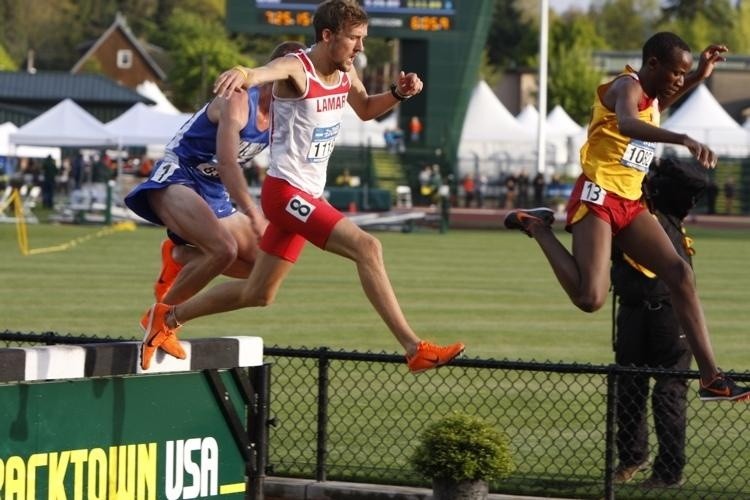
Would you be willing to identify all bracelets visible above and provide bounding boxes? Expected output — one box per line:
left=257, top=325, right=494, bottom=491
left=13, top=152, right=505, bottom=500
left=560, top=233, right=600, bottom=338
left=388, top=83, right=412, bottom=103
left=232, top=65, right=250, bottom=80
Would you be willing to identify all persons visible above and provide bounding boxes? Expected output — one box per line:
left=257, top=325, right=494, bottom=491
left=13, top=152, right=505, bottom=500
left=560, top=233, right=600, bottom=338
left=336, top=167, right=361, bottom=189
left=0, top=150, right=153, bottom=210
left=705, top=174, right=735, bottom=217
left=382, top=116, right=425, bottom=156
left=417, top=161, right=562, bottom=211
left=241, top=160, right=266, bottom=188
left=609, top=156, right=711, bottom=492
left=124, top=41, right=309, bottom=333
left=503, top=32, right=749, bottom=403
left=140, top=1, right=466, bottom=374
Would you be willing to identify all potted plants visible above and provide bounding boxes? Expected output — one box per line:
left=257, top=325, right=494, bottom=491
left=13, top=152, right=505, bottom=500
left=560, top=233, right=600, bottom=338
left=403, top=410, right=521, bottom=500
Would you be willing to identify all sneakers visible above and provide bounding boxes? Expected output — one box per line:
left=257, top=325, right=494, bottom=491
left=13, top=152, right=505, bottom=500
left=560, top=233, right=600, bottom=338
left=612, top=451, right=653, bottom=484
left=504, top=206, right=556, bottom=238
left=154, top=236, right=183, bottom=302
left=638, top=476, right=681, bottom=492
left=698, top=374, right=750, bottom=402
left=140, top=302, right=188, bottom=371
left=406, top=340, right=465, bottom=376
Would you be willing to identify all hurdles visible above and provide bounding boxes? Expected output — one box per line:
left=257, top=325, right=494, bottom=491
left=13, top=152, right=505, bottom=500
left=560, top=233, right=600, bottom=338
left=0, top=334, right=270, bottom=500
